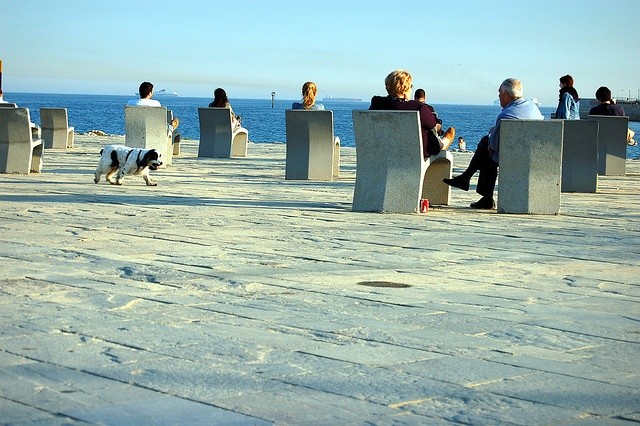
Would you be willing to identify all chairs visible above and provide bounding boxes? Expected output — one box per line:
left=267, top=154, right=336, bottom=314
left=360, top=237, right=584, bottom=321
left=198, top=107, right=248, bottom=158
left=588, top=114, right=629, bottom=177
left=285, top=110, right=341, bottom=182
left=0, top=107, right=44, bottom=175
left=349, top=109, right=454, bottom=214
left=40, top=107, right=74, bottom=149
left=124, top=105, right=172, bottom=166
left=560, top=118, right=599, bottom=194
left=497, top=118, right=565, bottom=216
left=1, top=103, right=41, bottom=141
left=167, top=110, right=181, bottom=156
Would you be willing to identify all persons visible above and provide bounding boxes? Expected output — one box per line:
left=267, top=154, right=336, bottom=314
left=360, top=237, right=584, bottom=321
left=443, top=77, right=545, bottom=209
left=292, top=81, right=325, bottom=110
left=589, top=87, right=638, bottom=147
left=368, top=70, right=455, bottom=157
left=458, top=137, right=466, bottom=150
left=208, top=88, right=241, bottom=132
left=414, top=89, right=444, bottom=136
left=556, top=75, right=580, bottom=120
left=127, top=82, right=179, bottom=134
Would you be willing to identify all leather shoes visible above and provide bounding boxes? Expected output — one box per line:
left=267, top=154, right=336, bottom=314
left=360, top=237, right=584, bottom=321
left=443, top=177, right=469, bottom=191
left=470, top=198, right=495, bottom=208
left=441, top=127, right=454, bottom=150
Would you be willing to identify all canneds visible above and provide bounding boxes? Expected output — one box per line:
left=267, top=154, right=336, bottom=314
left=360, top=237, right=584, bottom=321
left=421, top=199, right=429, bottom=213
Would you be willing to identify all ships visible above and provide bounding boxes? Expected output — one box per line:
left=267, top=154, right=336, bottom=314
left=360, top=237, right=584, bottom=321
left=568, top=94, right=634, bottom=127
left=322, top=93, right=365, bottom=108
left=135, top=88, right=178, bottom=96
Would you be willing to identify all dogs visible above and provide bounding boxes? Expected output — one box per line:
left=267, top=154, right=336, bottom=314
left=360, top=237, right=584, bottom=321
left=94, top=144, right=163, bottom=185
